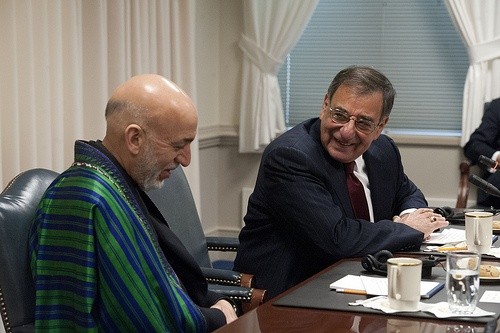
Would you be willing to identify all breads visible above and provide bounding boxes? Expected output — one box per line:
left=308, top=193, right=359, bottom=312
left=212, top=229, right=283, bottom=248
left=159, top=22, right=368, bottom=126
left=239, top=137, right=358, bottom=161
left=493, top=221, right=500, bottom=229
left=479, top=264, right=500, bottom=277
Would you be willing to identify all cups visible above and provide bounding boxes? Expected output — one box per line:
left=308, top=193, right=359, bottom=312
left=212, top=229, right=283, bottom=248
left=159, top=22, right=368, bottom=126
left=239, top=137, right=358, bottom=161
left=445, top=250, right=481, bottom=315
left=465, top=212, right=493, bottom=254
left=387, top=258, right=422, bottom=311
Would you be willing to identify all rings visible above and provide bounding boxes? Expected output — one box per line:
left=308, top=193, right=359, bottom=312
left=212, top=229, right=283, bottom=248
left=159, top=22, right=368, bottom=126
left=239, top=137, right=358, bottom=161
left=429, top=216, right=437, bottom=222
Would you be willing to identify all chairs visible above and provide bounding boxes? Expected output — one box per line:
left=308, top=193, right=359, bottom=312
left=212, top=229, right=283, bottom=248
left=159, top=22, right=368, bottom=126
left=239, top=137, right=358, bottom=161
left=455, top=162, right=479, bottom=209
left=0, top=167, right=271, bottom=333
left=143, top=161, right=257, bottom=288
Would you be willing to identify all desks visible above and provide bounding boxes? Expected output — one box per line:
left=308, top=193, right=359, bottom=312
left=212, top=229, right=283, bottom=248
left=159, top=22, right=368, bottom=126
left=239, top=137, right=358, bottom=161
left=212, top=208, right=500, bottom=333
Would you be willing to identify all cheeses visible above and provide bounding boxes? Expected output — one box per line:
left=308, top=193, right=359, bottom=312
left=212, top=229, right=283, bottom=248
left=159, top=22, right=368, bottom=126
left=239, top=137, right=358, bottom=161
left=439, top=242, right=467, bottom=251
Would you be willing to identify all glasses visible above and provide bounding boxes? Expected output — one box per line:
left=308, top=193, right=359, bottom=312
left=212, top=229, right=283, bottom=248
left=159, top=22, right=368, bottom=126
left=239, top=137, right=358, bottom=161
left=328, top=100, right=383, bottom=135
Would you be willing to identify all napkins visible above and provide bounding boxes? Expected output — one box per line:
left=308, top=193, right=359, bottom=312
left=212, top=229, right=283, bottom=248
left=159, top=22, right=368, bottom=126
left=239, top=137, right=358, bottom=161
left=421, top=301, right=497, bottom=319
left=348, top=294, right=427, bottom=314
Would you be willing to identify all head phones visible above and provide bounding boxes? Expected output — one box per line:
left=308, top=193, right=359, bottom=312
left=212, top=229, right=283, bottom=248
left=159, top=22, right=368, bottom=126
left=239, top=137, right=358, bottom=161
left=433, top=206, right=454, bottom=224
left=361, top=250, right=438, bottom=276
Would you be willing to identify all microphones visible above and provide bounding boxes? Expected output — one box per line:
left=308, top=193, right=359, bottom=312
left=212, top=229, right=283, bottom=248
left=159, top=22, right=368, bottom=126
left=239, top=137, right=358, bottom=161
left=478, top=155, right=500, bottom=169
left=468, top=174, right=500, bottom=197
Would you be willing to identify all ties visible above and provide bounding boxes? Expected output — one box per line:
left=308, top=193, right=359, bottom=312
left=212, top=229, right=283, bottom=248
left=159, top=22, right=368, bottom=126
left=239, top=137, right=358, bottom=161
left=344, top=161, right=370, bottom=223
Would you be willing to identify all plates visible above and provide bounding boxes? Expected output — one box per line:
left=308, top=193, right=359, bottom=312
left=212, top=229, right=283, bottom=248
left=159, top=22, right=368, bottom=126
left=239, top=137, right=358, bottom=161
left=492, top=220, right=500, bottom=232
left=479, top=267, right=500, bottom=282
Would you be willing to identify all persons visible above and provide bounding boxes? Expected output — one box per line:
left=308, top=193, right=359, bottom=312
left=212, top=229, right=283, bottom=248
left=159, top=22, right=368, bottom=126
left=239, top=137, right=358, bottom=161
left=462, top=96, right=500, bottom=211
left=232, top=64, right=449, bottom=293
left=25, top=74, right=240, bottom=333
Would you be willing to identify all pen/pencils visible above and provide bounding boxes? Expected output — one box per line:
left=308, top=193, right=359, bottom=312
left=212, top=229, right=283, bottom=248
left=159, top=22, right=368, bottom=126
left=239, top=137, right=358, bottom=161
left=423, top=247, right=467, bottom=252
left=335, top=288, right=366, bottom=294
